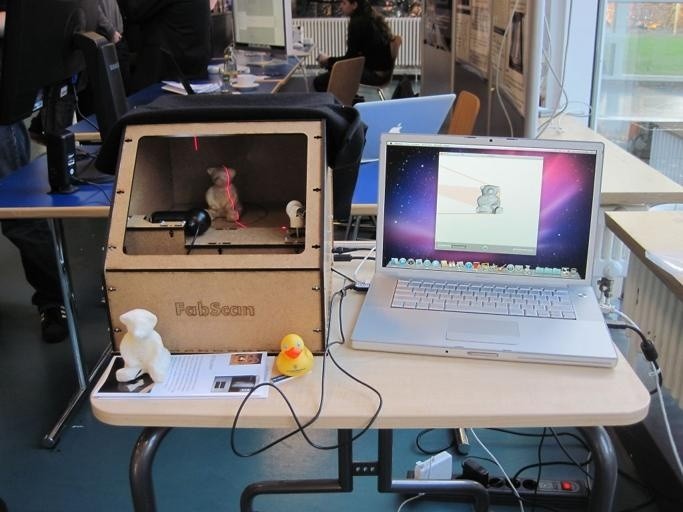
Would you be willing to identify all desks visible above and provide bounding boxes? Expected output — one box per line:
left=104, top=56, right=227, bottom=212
left=91, top=241, right=651, bottom=512
left=604, top=210, right=683, bottom=300
left=536, top=116, right=683, bottom=205
left=166, top=38, right=320, bottom=92
left=0, top=145, right=116, bottom=449
left=65, top=73, right=282, bottom=141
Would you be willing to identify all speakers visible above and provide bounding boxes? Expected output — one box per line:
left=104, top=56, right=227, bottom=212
left=46, top=130, right=79, bottom=196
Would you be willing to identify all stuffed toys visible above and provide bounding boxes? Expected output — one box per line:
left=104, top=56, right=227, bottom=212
left=205, top=166, right=242, bottom=222
left=115, top=309, right=172, bottom=384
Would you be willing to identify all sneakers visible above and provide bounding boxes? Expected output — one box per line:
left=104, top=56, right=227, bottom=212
left=35, top=301, right=75, bottom=342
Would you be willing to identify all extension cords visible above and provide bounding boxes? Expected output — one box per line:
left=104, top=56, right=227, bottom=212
left=405, top=470, right=589, bottom=506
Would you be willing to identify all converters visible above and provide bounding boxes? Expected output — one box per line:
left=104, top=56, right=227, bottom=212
left=415, top=450, right=452, bottom=480
left=463, top=460, right=489, bottom=488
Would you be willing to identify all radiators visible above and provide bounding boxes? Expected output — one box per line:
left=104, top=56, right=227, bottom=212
left=293, top=17, right=422, bottom=82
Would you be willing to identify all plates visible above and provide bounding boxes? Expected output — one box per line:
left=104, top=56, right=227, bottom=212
left=231, top=83, right=260, bottom=92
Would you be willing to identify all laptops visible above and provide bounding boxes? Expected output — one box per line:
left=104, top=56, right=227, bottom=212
left=350, top=132, right=619, bottom=368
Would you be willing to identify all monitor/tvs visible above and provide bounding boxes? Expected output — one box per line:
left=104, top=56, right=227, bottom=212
left=231, top=0, right=288, bottom=56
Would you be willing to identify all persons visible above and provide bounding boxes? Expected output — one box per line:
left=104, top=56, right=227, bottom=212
left=314, top=0, right=395, bottom=104
left=64, top=0, right=125, bottom=54
left=0, top=0, right=80, bottom=345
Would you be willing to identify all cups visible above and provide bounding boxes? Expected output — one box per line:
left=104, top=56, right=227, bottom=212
left=236, top=74, right=258, bottom=86
left=292, top=25, right=303, bottom=46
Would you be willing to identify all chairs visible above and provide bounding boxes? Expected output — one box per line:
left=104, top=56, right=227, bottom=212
left=445, top=90, right=480, bottom=135
left=342, top=203, right=377, bottom=240
left=356, top=35, right=404, bottom=101
left=323, top=57, right=366, bottom=106
